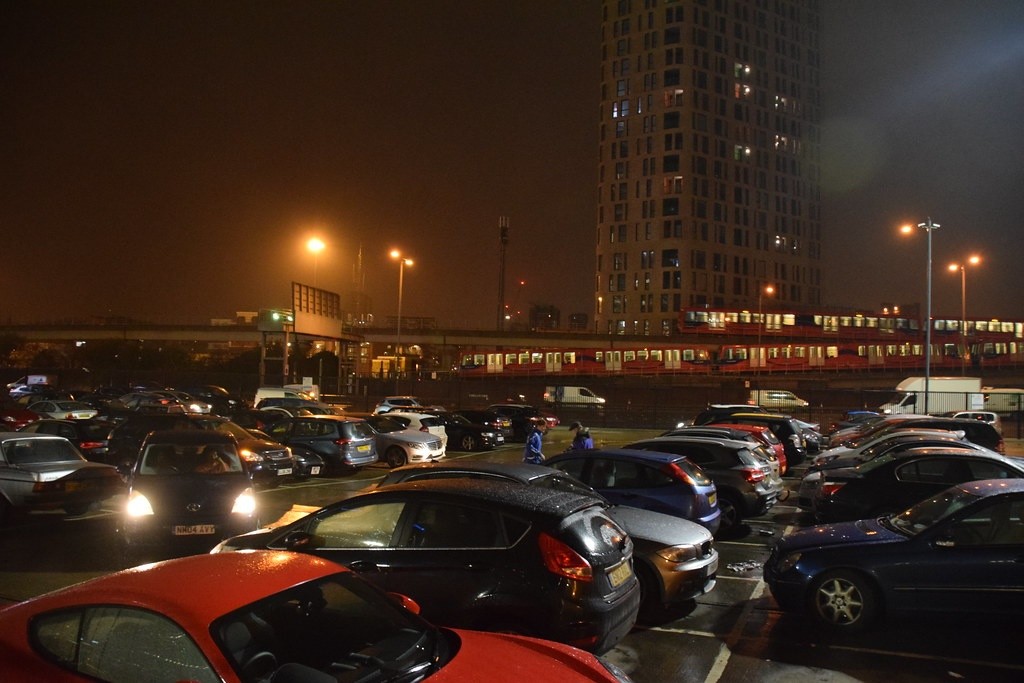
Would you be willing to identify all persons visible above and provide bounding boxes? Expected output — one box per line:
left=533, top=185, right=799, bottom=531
left=523, top=420, right=547, bottom=463
left=562, top=422, right=594, bottom=453
left=196, top=450, right=230, bottom=474
left=146, top=451, right=185, bottom=475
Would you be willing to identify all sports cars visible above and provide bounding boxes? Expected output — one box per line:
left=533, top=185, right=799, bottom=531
left=0, top=549, right=630, bottom=683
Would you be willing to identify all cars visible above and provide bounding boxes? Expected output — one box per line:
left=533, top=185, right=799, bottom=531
left=797, top=411, right=1024, bottom=516
left=253, top=384, right=561, bottom=477
left=115, top=432, right=263, bottom=551
left=0, top=374, right=293, bottom=523
left=763, top=479, right=1024, bottom=633
left=536, top=448, right=721, bottom=536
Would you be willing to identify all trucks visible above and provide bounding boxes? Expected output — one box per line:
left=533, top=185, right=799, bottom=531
left=981, top=387, right=1024, bottom=418
left=876, top=377, right=981, bottom=418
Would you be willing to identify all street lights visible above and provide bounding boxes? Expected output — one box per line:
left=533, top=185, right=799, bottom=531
left=898, top=217, right=940, bottom=415
left=947, top=254, right=981, bottom=376
left=756, top=283, right=775, bottom=407
left=388, top=248, right=415, bottom=396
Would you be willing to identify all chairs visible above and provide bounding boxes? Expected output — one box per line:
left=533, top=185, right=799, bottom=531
left=592, top=461, right=611, bottom=484
left=981, top=507, right=1010, bottom=539
left=220, top=621, right=262, bottom=670
left=310, top=422, right=317, bottom=434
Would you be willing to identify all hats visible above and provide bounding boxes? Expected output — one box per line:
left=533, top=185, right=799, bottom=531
left=569, top=422, right=580, bottom=431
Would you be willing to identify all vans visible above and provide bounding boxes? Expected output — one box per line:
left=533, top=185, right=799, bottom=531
left=544, top=386, right=606, bottom=410
left=952, top=411, right=1002, bottom=437
left=748, top=390, right=809, bottom=411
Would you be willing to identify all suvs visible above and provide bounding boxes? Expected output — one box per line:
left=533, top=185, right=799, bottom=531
left=653, top=404, right=823, bottom=478
left=373, top=460, right=718, bottom=604
left=210, top=479, right=641, bottom=657
left=620, top=436, right=785, bottom=536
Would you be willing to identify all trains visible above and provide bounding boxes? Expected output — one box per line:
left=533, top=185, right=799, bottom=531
left=675, top=306, right=1024, bottom=341
left=451, top=335, right=1024, bottom=378
